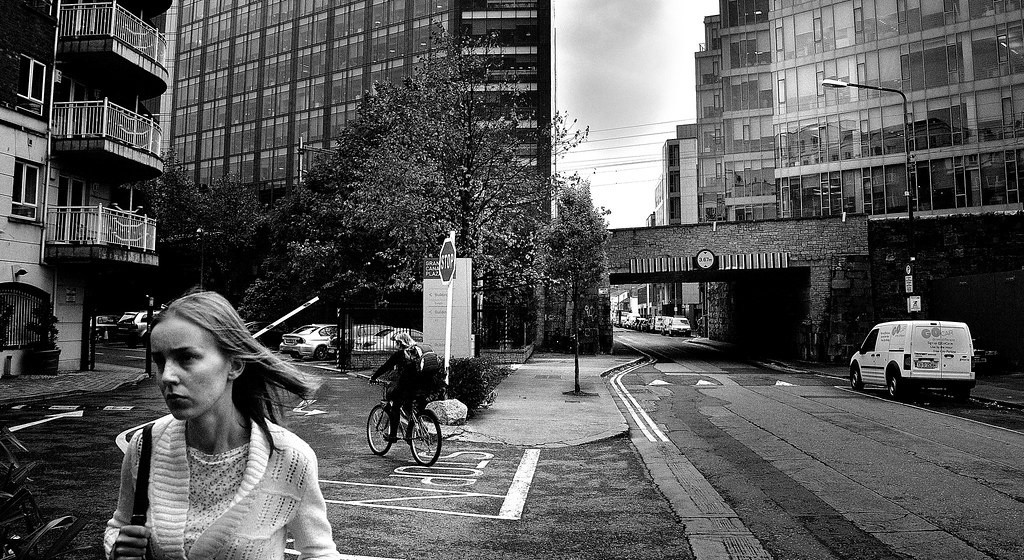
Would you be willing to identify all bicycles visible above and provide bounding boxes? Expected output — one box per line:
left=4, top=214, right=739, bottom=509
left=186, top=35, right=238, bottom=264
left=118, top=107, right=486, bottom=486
left=367, top=380, right=442, bottom=466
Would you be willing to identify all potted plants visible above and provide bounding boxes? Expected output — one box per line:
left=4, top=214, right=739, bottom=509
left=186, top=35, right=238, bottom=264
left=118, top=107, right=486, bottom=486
left=25, top=298, right=61, bottom=376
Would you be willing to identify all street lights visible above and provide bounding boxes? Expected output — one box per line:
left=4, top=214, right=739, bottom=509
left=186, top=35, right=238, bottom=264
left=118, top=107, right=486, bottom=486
left=822, top=79, right=920, bottom=322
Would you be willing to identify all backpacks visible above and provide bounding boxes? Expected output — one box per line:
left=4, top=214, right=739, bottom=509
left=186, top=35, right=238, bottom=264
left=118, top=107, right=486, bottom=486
left=402, top=347, right=441, bottom=393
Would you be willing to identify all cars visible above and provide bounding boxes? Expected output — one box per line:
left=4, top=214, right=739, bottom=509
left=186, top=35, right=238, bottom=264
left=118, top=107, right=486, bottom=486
left=971, top=324, right=1024, bottom=372
left=244, top=321, right=297, bottom=351
left=613, top=311, right=691, bottom=337
left=280, top=323, right=424, bottom=361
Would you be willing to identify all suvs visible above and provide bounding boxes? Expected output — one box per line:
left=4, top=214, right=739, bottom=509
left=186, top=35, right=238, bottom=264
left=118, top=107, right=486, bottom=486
left=117, top=310, right=160, bottom=347
left=88, top=314, right=121, bottom=343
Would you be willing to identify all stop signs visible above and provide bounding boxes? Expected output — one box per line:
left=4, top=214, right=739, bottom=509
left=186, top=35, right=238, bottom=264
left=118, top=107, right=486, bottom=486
left=439, top=238, right=455, bottom=285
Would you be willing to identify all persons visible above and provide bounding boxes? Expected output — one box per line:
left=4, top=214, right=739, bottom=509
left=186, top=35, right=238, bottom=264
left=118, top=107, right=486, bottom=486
left=104, top=291, right=340, bottom=560
left=370, top=333, right=438, bottom=443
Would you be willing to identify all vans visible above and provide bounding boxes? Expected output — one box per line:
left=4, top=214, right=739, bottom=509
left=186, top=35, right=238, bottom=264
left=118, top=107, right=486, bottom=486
left=849, top=323, right=978, bottom=404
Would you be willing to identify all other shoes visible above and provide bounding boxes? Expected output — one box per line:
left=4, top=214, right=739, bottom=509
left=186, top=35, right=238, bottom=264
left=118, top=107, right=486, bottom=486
left=383, top=433, right=397, bottom=443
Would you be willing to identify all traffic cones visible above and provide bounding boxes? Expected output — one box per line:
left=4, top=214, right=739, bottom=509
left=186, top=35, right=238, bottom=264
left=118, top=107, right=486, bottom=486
left=103, top=327, right=110, bottom=346
